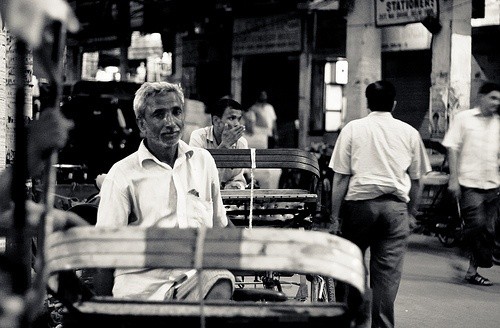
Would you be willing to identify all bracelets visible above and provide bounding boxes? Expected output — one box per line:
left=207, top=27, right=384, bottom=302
left=329, top=218, right=338, bottom=224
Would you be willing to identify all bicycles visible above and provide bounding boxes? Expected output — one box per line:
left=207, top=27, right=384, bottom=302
left=283, top=141, right=335, bottom=228
left=413, top=145, right=465, bottom=248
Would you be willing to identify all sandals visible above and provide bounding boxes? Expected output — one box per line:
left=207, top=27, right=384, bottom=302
left=463, top=272, right=493, bottom=286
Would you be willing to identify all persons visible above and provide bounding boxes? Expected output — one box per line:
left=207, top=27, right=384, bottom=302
left=189, top=98, right=248, bottom=190
left=242, top=90, right=280, bottom=150
left=431, top=112, right=443, bottom=137
left=328, top=81, right=432, bottom=328
left=95, top=82, right=238, bottom=301
left=441, top=81, right=500, bottom=286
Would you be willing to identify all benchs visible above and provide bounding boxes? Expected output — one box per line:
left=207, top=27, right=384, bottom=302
left=42, top=226, right=370, bottom=328
left=208, top=148, right=321, bottom=229
